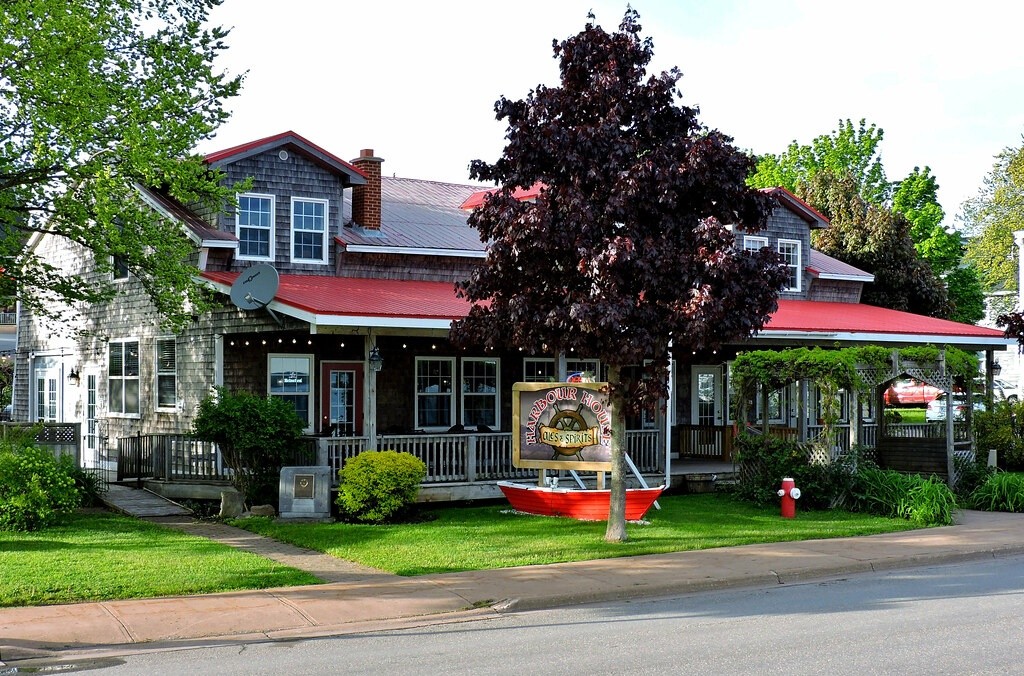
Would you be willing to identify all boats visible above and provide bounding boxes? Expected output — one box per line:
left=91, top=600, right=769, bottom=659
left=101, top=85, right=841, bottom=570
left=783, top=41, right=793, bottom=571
left=496, top=479, right=667, bottom=520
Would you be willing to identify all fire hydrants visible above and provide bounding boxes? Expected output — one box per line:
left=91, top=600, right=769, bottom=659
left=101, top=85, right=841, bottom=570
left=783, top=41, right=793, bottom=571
left=777, top=478, right=801, bottom=519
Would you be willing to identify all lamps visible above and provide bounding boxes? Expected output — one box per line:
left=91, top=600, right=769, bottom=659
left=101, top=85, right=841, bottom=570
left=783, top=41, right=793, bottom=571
left=66, top=367, right=78, bottom=385
left=369, top=346, right=384, bottom=372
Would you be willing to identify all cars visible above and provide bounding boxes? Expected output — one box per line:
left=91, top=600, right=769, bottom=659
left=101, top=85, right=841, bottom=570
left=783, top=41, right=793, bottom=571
left=882, top=377, right=968, bottom=409
left=927, top=388, right=1005, bottom=423
